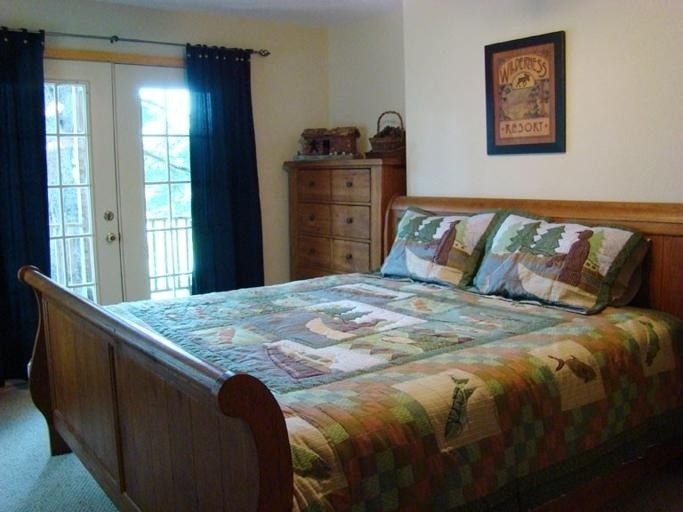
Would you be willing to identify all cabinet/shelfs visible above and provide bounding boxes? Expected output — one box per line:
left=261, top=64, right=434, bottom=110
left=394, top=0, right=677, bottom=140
left=283, top=156, right=407, bottom=280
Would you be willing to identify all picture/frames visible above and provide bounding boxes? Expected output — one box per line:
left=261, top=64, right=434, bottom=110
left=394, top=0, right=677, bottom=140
left=484, top=30, right=565, bottom=155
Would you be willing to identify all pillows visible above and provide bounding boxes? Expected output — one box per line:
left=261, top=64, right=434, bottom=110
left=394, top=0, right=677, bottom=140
left=380, top=207, right=652, bottom=316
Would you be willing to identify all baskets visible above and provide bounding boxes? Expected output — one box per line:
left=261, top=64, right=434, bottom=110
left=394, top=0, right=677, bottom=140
left=368, top=111, right=406, bottom=153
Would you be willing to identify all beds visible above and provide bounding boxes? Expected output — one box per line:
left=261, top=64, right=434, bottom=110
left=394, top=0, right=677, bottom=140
left=17, top=196, right=683, bottom=510
